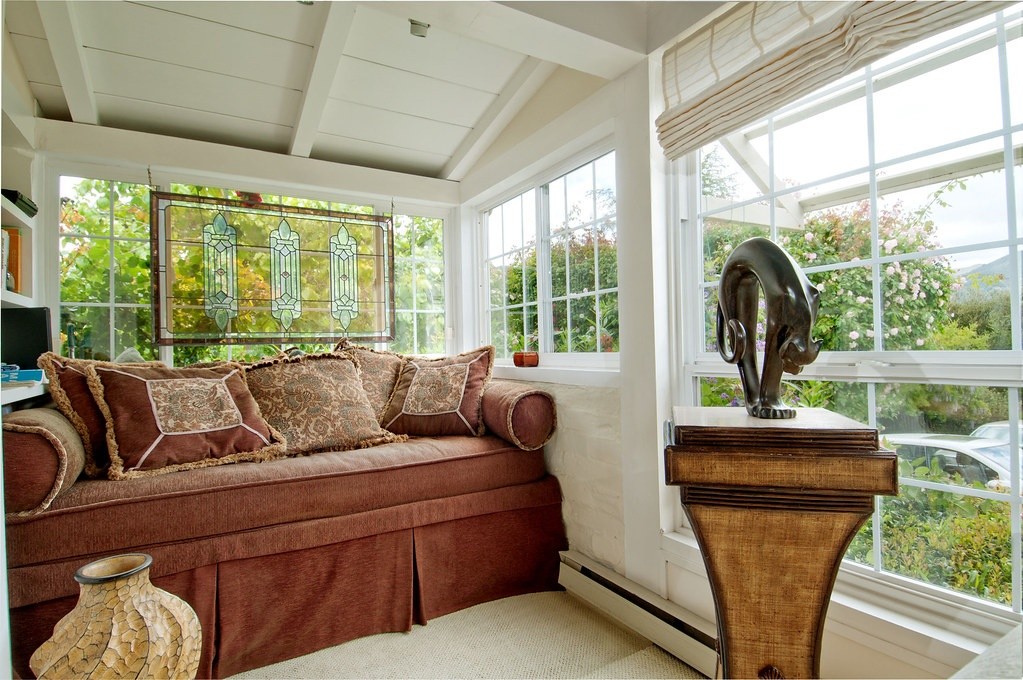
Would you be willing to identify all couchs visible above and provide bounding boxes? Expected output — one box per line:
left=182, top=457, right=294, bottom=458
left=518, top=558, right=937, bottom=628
left=1, top=336, right=569, bottom=680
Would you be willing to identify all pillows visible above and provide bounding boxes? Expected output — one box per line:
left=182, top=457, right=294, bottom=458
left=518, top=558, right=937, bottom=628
left=37, top=351, right=168, bottom=478
left=379, top=344, right=496, bottom=438
left=84, top=362, right=289, bottom=480
left=332, top=337, right=404, bottom=425
left=244, top=348, right=409, bottom=458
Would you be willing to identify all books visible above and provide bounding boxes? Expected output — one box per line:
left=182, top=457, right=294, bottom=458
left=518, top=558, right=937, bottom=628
left=1, top=225, right=23, bottom=293
left=0, top=369, right=49, bottom=387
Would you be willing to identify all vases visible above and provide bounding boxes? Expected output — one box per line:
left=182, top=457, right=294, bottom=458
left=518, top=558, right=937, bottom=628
left=29, top=553, right=202, bottom=680
left=513, top=352, right=539, bottom=367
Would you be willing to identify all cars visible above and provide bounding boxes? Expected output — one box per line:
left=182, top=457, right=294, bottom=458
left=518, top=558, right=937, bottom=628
left=877, top=418, right=1023, bottom=498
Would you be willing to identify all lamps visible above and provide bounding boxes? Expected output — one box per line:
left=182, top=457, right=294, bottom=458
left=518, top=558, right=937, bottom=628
left=408, top=18, right=430, bottom=37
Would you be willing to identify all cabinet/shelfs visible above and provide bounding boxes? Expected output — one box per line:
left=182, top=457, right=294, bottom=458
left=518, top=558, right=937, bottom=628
left=0, top=194, right=52, bottom=406
